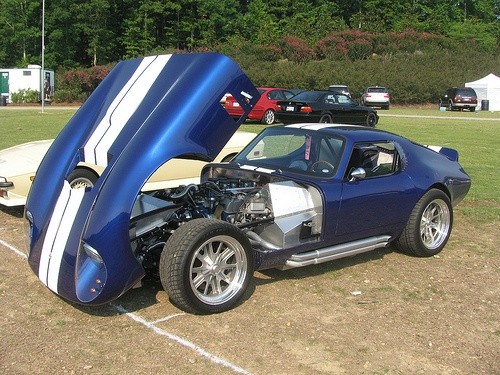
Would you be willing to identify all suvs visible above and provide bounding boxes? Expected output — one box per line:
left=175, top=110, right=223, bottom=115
left=360, top=85, right=389, bottom=109
left=326, top=85, right=352, bottom=105
left=439, top=86, right=478, bottom=112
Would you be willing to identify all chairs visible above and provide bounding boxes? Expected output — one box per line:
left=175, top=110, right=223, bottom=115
left=328, top=99, right=335, bottom=104
left=345, top=148, right=379, bottom=180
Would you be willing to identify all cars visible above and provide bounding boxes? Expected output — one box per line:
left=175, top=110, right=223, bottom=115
left=0, top=130, right=269, bottom=207
left=25, top=52, right=472, bottom=315
left=277, top=90, right=380, bottom=128
left=225, top=88, right=295, bottom=124
